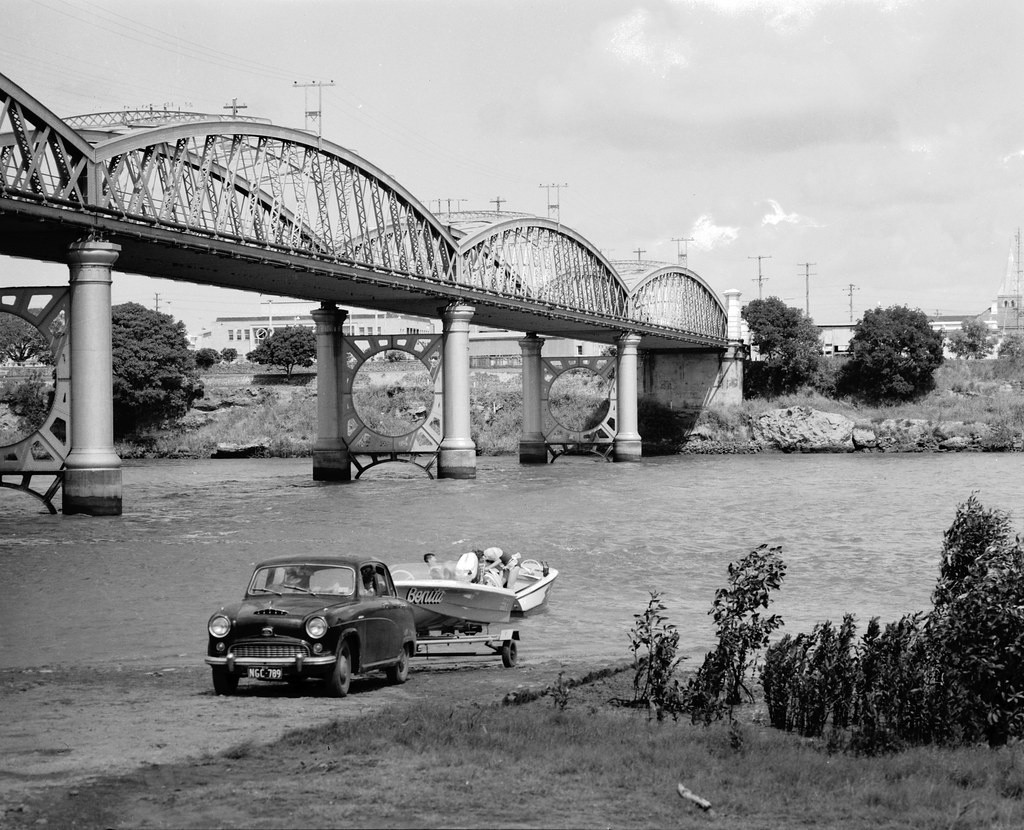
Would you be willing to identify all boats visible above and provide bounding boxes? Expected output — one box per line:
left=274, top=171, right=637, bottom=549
left=374, top=562, right=559, bottom=628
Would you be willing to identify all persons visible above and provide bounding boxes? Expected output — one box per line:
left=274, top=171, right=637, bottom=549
left=484, top=547, right=521, bottom=589
left=423, top=553, right=444, bottom=579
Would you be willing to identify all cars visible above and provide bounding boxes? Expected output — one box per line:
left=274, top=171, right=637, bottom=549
left=204, top=553, right=419, bottom=698
